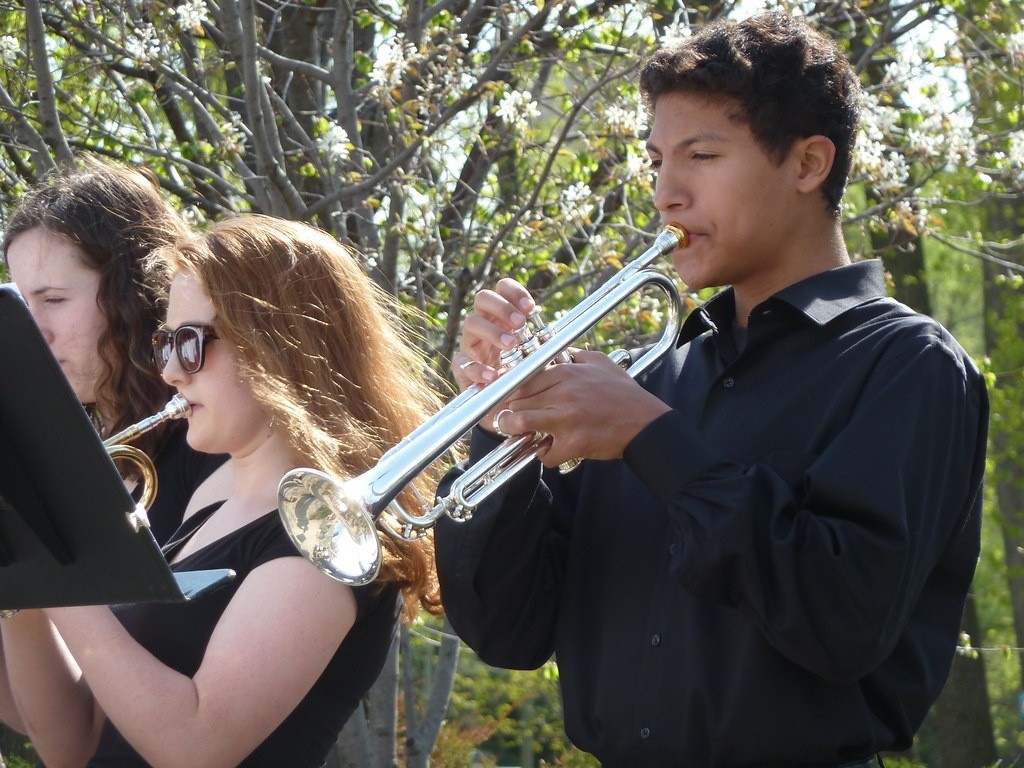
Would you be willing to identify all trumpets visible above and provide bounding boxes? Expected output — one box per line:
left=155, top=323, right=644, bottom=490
left=274, top=221, right=690, bottom=589
left=102, top=390, right=193, bottom=515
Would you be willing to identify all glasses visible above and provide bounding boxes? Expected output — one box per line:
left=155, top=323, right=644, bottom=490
left=151, top=320, right=219, bottom=375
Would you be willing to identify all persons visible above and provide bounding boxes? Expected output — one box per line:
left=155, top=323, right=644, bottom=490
left=0, top=215, right=446, bottom=768
left=0, top=168, right=230, bottom=736
left=433, top=10, right=990, bottom=768
